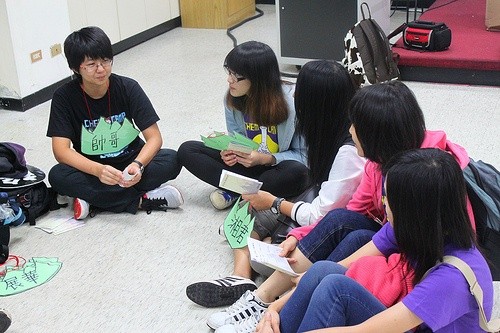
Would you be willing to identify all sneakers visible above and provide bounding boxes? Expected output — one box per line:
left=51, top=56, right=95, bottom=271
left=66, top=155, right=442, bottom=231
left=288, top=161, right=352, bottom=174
left=74, top=197, right=98, bottom=220
left=209, top=189, right=241, bottom=211
left=214, top=309, right=279, bottom=333
left=186, top=276, right=258, bottom=308
left=140, top=185, right=184, bottom=214
left=206, top=290, right=279, bottom=331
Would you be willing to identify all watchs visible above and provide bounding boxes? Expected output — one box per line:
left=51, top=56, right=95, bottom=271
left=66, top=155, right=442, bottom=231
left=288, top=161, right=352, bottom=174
left=270, top=197, right=285, bottom=215
left=133, top=160, right=145, bottom=173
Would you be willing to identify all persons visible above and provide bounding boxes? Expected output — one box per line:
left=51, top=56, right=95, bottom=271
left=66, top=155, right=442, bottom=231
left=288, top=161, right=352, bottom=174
left=46, top=25, right=184, bottom=220
left=205, top=81, right=493, bottom=333
left=187, top=59, right=367, bottom=306
left=178, top=42, right=314, bottom=209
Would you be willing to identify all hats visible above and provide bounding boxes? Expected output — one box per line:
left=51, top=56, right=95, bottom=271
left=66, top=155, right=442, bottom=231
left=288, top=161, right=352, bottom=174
left=0, top=142, right=45, bottom=189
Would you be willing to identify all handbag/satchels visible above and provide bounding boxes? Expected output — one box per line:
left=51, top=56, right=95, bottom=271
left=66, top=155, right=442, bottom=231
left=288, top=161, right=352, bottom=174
left=386, top=20, right=451, bottom=51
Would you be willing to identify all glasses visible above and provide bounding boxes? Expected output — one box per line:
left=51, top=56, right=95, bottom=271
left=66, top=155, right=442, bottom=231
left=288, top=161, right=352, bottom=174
left=80, top=58, right=111, bottom=72
left=223, top=65, right=246, bottom=83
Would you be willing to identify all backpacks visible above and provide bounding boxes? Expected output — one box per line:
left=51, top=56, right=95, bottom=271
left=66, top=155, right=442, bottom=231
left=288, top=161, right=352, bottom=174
left=341, top=2, right=401, bottom=88
left=462, top=157, right=500, bottom=281
left=0, top=182, right=51, bottom=264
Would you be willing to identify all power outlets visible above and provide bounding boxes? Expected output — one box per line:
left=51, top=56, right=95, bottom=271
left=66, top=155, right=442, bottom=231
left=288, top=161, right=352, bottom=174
left=30, top=50, right=42, bottom=63
